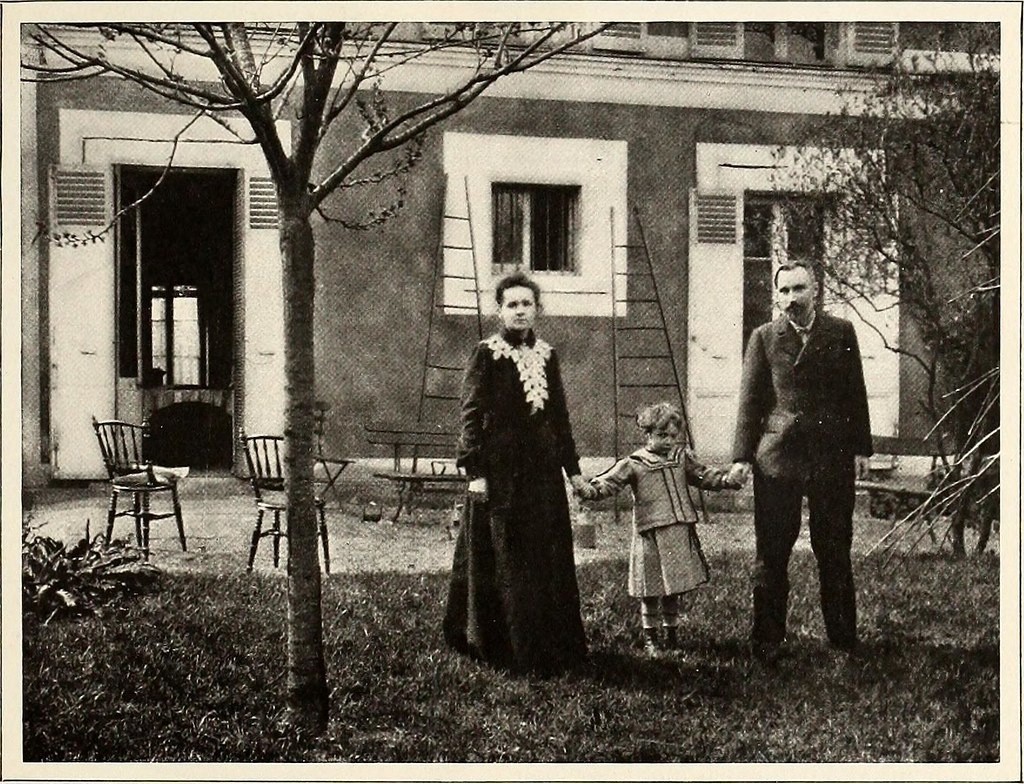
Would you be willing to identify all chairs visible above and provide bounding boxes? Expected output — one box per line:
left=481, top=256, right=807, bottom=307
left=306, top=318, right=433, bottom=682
left=239, top=427, right=330, bottom=575
left=91, top=415, right=187, bottom=561
left=315, top=401, right=356, bottom=514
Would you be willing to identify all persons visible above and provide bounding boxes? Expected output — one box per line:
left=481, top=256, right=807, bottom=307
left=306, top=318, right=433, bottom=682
left=580, top=402, right=751, bottom=658
left=729, top=257, right=875, bottom=660
left=439, top=272, right=592, bottom=678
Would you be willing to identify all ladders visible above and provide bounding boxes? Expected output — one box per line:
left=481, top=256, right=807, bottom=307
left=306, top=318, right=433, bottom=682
left=610, top=206, right=709, bottom=527
left=406, top=172, right=484, bottom=517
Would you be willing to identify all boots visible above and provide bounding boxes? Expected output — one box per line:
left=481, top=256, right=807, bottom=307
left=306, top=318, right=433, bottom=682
left=663, top=626, right=684, bottom=658
left=644, top=627, right=663, bottom=659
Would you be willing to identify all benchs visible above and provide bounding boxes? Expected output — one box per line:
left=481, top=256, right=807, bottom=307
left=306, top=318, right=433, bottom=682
left=364, top=426, right=466, bottom=521
